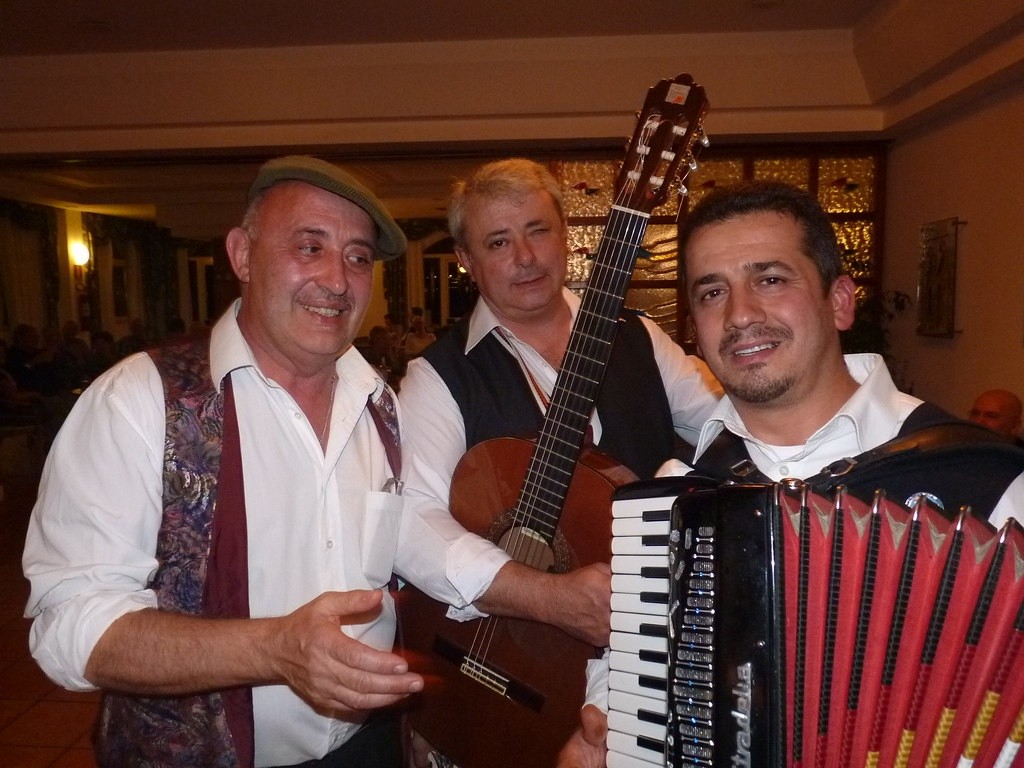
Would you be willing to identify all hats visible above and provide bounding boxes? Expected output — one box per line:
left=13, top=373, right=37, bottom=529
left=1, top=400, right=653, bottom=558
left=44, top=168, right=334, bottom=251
left=247, top=155, right=407, bottom=261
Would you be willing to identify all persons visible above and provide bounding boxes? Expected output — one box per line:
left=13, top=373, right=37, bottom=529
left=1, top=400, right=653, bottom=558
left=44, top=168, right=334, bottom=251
left=0, top=156, right=1024, bottom=768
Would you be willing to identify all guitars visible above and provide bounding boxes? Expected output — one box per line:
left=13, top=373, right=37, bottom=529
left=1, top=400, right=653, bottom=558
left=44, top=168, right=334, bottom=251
left=387, top=69, right=713, bottom=767
